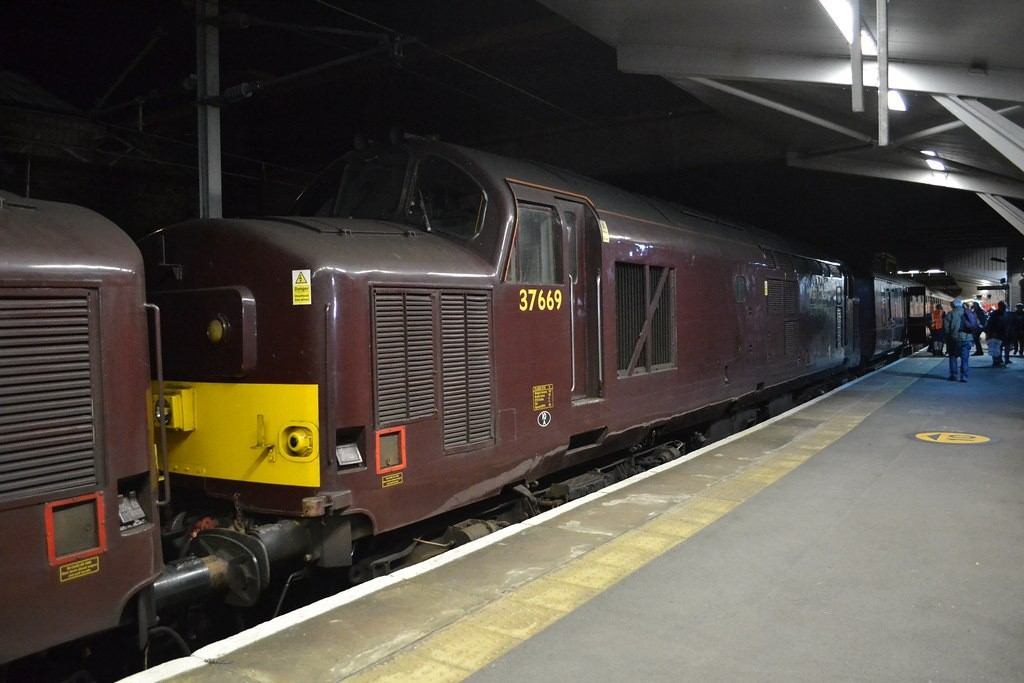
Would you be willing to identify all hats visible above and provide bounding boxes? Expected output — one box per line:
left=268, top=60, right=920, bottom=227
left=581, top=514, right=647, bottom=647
left=953, top=298, right=962, bottom=306
left=936, top=304, right=942, bottom=308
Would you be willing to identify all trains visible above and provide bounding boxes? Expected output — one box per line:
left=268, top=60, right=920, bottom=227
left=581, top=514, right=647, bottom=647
left=1, top=126, right=960, bottom=673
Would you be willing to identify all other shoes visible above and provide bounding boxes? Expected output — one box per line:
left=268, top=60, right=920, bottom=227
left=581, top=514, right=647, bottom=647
left=927, top=349, right=932, bottom=352
left=993, top=363, right=1002, bottom=367
left=947, top=375, right=956, bottom=380
left=971, top=350, right=983, bottom=355
left=1014, top=348, right=1018, bottom=354
left=1005, top=360, right=1012, bottom=363
left=961, top=376, right=968, bottom=382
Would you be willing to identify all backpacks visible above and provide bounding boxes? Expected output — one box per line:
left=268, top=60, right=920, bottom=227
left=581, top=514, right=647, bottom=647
left=925, top=312, right=932, bottom=326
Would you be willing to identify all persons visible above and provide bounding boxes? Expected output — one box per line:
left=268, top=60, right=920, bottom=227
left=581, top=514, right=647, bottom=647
left=943, top=299, right=980, bottom=383
left=966, top=300, right=1024, bottom=368
left=927, top=304, right=948, bottom=357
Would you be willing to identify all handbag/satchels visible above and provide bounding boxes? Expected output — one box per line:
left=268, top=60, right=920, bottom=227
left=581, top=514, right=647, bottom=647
left=958, top=308, right=980, bottom=334
left=986, top=338, right=1002, bottom=357
